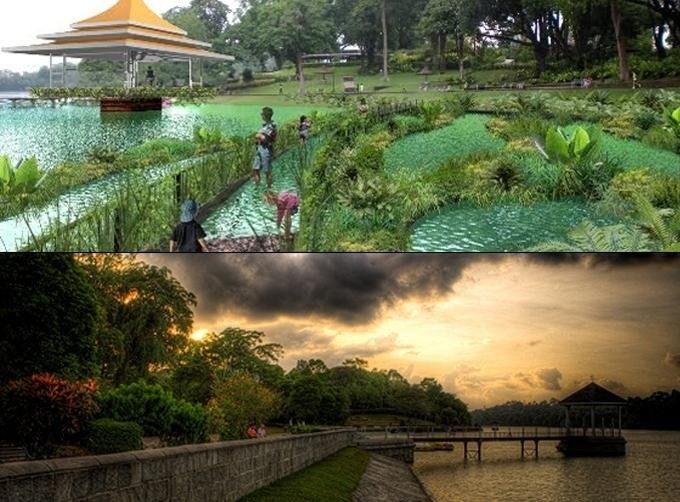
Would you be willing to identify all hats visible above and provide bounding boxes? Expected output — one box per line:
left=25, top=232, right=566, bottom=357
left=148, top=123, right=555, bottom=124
left=180, top=200, right=200, bottom=223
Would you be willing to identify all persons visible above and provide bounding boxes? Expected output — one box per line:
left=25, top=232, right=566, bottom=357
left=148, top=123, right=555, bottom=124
left=297, top=115, right=312, bottom=144
left=252, top=106, right=276, bottom=188
left=357, top=97, right=369, bottom=118
left=144, top=62, right=643, bottom=96
left=169, top=198, right=209, bottom=252
left=259, top=188, right=301, bottom=240
